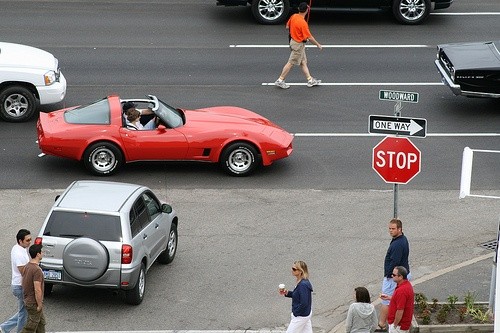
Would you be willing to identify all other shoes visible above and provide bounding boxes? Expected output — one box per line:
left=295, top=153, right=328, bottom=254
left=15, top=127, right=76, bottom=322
left=307, top=78, right=322, bottom=88
left=274, top=78, right=291, bottom=89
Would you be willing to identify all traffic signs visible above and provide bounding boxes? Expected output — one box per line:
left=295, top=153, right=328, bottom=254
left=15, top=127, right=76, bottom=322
left=368, top=115, right=427, bottom=139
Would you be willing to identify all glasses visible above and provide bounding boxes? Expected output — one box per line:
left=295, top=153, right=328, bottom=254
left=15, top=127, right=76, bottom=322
left=292, top=266, right=299, bottom=271
left=392, top=272, right=399, bottom=277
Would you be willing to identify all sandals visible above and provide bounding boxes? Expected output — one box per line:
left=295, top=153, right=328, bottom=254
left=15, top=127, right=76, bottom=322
left=374, top=323, right=387, bottom=332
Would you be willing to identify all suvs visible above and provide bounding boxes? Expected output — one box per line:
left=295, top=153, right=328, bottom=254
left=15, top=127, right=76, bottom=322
left=0, top=42, right=67, bottom=124
left=32, top=180, right=179, bottom=306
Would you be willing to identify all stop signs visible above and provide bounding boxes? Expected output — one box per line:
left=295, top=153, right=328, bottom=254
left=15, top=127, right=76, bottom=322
left=372, top=135, right=422, bottom=185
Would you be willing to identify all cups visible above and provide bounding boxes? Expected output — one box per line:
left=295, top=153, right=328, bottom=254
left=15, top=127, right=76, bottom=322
left=279, top=284, right=285, bottom=296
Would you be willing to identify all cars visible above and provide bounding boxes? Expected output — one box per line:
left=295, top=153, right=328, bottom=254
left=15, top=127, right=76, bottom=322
left=216, top=0, right=454, bottom=26
left=433, top=39, right=500, bottom=100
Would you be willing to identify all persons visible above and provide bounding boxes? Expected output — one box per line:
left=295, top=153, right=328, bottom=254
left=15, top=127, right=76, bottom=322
left=345, top=286, right=379, bottom=333
left=123, top=100, right=154, bottom=127
left=20, top=243, right=46, bottom=333
left=378, top=266, right=414, bottom=333
left=125, top=109, right=141, bottom=130
left=371, top=219, right=410, bottom=332
left=274, top=1, right=324, bottom=89
left=279, top=260, right=315, bottom=333
left=0, top=228, right=35, bottom=333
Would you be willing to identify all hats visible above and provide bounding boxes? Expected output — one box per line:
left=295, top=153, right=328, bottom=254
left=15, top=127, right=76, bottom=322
left=123, top=103, right=138, bottom=111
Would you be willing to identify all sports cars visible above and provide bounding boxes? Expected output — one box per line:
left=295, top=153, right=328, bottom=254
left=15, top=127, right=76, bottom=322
left=35, top=93, right=295, bottom=178
